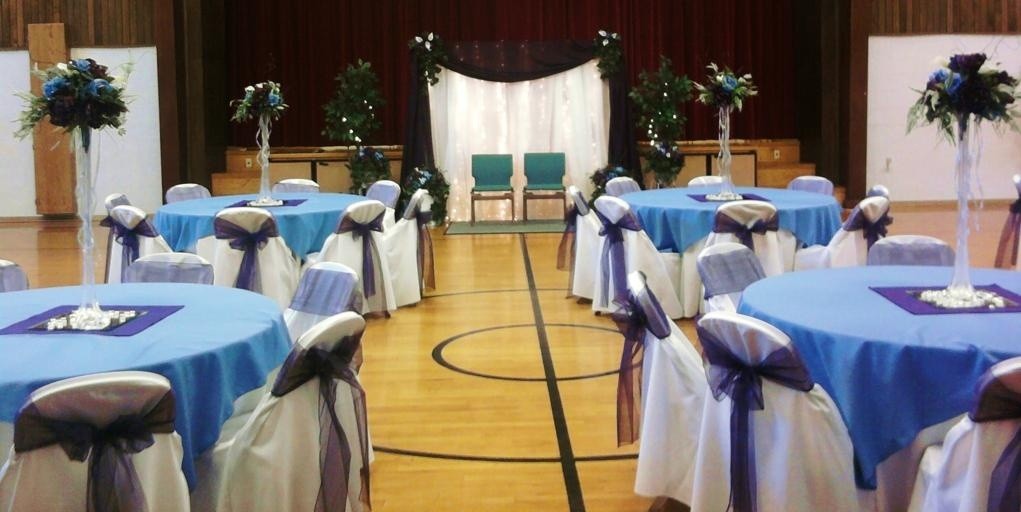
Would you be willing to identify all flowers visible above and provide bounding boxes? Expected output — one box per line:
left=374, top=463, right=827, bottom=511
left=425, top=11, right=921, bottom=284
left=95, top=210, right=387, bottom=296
left=5, top=34, right=143, bottom=166
left=891, top=32, right=1021, bottom=160
left=686, top=57, right=760, bottom=121
left=225, top=75, right=291, bottom=135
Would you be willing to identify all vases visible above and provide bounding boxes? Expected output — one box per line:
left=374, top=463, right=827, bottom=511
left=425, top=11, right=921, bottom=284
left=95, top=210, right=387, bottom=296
left=704, top=99, right=743, bottom=203
left=919, top=108, right=1000, bottom=312
left=63, top=123, right=116, bottom=330
left=246, top=108, right=285, bottom=208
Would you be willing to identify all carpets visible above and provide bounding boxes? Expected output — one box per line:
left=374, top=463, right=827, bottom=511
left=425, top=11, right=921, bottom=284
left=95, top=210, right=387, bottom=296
left=444, top=217, right=571, bottom=237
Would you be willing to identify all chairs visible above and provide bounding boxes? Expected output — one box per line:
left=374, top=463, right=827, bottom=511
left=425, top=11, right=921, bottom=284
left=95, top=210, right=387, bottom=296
left=464, top=151, right=517, bottom=227
left=521, top=149, right=569, bottom=225
